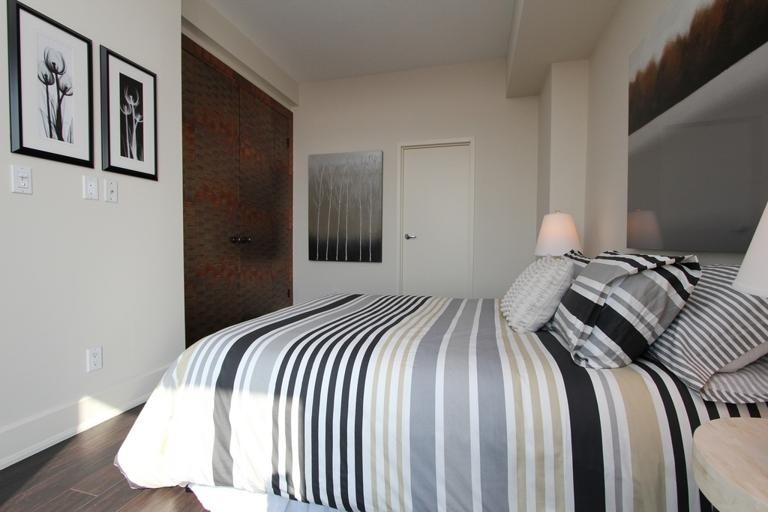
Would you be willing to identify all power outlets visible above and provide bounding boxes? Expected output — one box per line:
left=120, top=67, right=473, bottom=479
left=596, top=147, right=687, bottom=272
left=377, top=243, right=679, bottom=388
left=83, top=176, right=99, bottom=200
left=88, top=347, right=104, bottom=371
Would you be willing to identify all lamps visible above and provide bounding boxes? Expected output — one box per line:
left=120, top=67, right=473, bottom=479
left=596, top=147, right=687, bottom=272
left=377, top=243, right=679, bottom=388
left=730, top=202, right=768, bottom=297
left=535, top=212, right=581, bottom=257
left=626, top=210, right=664, bottom=251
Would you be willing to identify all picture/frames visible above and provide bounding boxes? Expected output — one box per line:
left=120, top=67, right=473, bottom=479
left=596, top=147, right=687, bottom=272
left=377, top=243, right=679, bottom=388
left=8, top=0, right=93, bottom=169
left=101, top=46, right=160, bottom=181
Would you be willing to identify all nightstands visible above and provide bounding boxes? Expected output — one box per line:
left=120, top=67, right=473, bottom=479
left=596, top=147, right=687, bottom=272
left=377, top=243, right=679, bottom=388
left=690, top=416, right=767, bottom=510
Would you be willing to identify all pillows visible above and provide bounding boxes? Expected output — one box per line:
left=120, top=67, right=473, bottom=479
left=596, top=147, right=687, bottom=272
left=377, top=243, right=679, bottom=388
left=564, top=248, right=592, bottom=279
left=550, top=252, right=703, bottom=372
left=645, top=265, right=768, bottom=405
left=500, top=257, right=569, bottom=334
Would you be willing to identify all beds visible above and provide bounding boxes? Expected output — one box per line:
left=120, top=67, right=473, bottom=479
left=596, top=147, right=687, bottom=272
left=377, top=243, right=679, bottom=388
left=111, top=294, right=768, bottom=512
